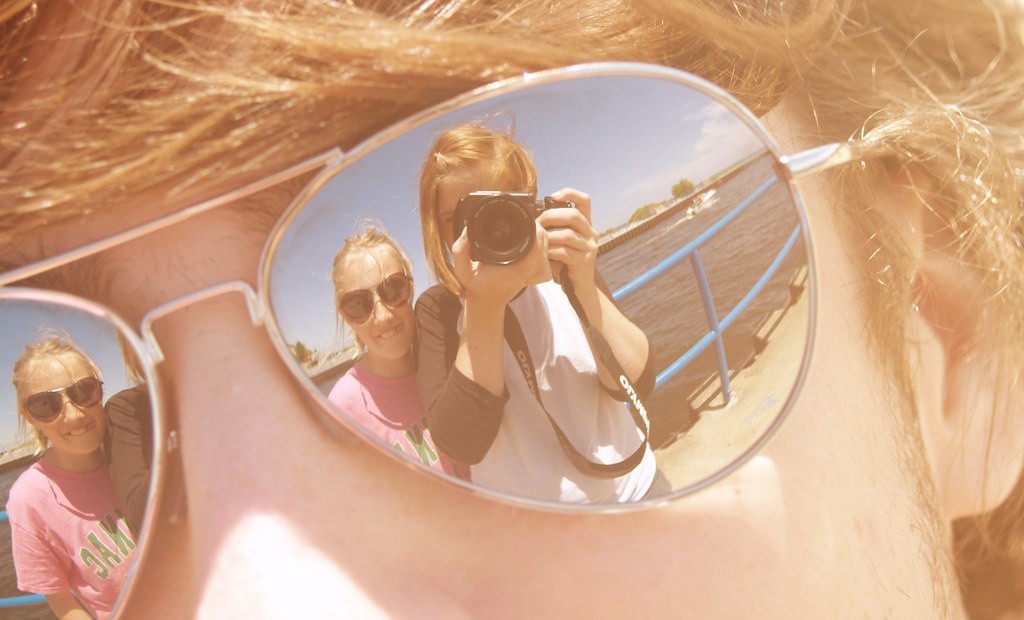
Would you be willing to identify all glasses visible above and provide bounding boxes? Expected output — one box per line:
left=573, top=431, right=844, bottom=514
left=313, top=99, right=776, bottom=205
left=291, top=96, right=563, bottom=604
left=339, top=272, right=413, bottom=322
left=24, top=377, right=104, bottom=423
left=0, top=60, right=968, bottom=620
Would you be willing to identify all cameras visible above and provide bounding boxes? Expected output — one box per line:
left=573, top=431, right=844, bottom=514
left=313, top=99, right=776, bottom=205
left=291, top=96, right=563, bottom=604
left=450, top=193, right=573, bottom=264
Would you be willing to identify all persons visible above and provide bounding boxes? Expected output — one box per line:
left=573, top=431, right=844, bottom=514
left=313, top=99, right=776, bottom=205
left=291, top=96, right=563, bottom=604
left=7, top=337, right=138, bottom=620
left=102, top=329, right=154, bottom=534
left=326, top=232, right=472, bottom=487
left=0, top=0, right=1024, bottom=620
left=415, top=109, right=670, bottom=507
